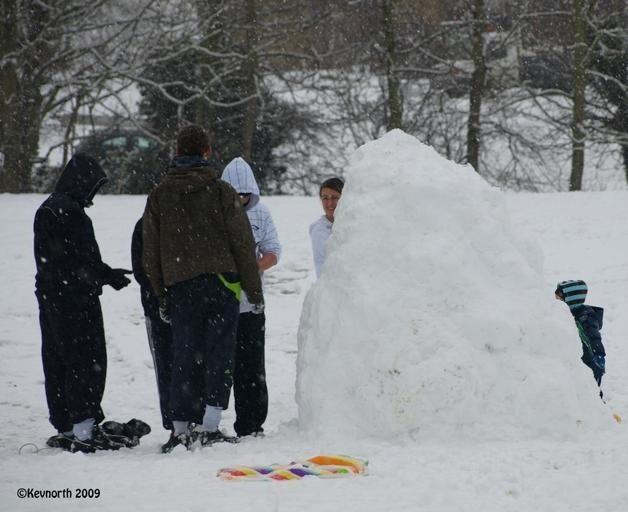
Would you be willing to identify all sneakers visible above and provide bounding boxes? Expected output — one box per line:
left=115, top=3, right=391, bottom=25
left=162, top=429, right=240, bottom=454
left=47, top=430, right=121, bottom=453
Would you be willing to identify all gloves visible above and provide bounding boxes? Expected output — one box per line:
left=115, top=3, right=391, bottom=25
left=252, top=302, right=264, bottom=314
left=106, top=269, right=132, bottom=290
left=159, top=303, right=171, bottom=325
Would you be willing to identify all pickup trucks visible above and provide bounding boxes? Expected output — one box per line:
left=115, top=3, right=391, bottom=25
left=396, top=11, right=592, bottom=98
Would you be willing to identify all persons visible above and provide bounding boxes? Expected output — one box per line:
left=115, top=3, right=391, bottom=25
left=309, top=178, right=344, bottom=279
left=34, top=152, right=132, bottom=453
left=555, top=279, right=606, bottom=399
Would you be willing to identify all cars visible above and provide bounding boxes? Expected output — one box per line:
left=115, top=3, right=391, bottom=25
left=70, top=129, right=169, bottom=195
